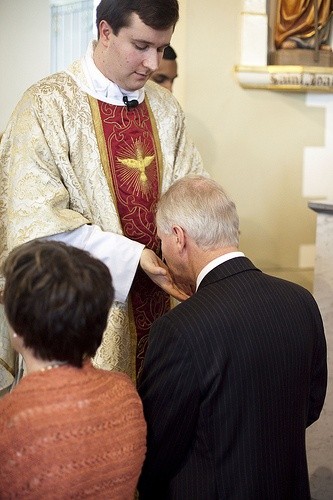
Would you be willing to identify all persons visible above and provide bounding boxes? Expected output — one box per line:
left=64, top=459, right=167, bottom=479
left=0, top=0, right=206, bottom=385
left=0, top=239, right=147, bottom=500
left=134, top=173, right=327, bottom=500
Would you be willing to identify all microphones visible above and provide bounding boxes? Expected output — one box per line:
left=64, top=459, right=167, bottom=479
left=123, top=96, right=138, bottom=107
